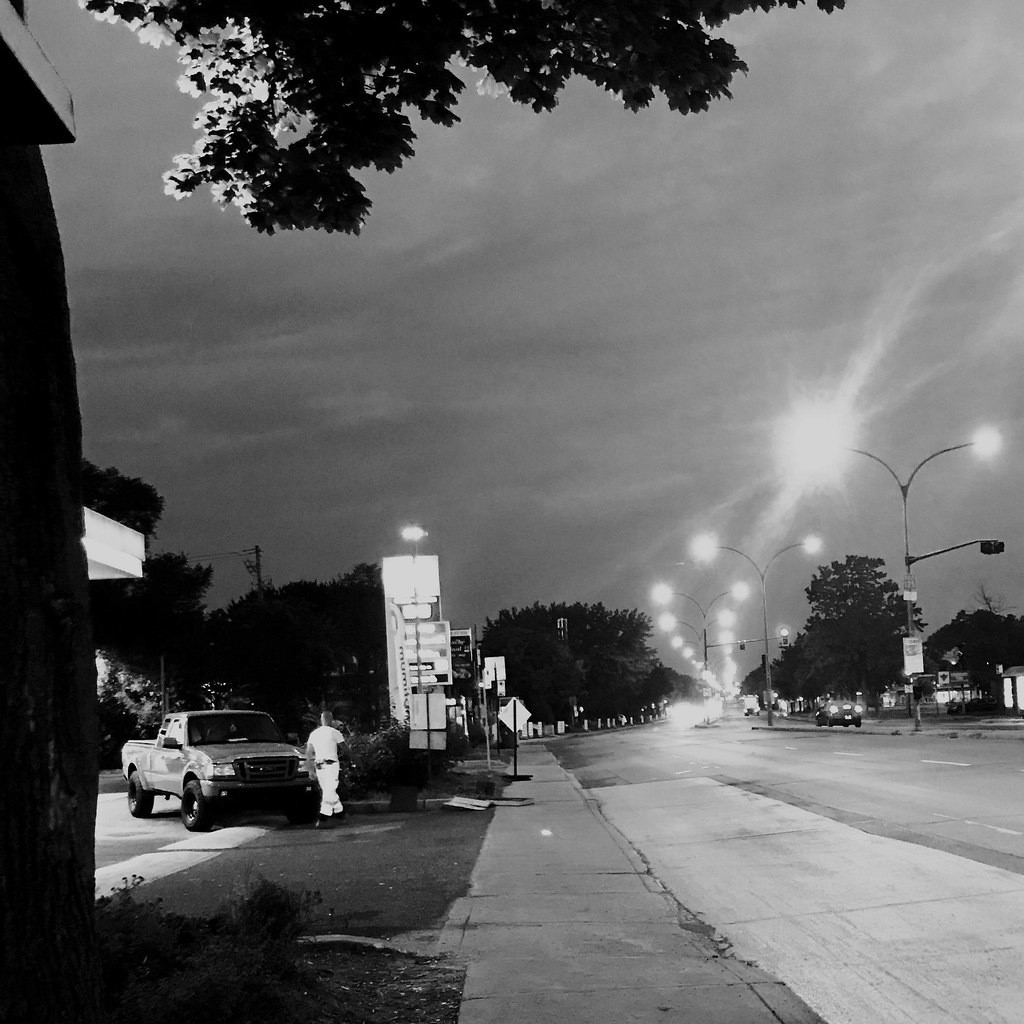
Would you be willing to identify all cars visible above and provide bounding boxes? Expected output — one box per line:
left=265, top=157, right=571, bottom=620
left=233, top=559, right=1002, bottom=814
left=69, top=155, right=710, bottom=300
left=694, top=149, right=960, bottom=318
left=815, top=699, right=864, bottom=728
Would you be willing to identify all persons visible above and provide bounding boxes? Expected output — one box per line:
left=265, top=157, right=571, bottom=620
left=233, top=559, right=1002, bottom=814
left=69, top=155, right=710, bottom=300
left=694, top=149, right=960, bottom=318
left=305, top=711, right=356, bottom=829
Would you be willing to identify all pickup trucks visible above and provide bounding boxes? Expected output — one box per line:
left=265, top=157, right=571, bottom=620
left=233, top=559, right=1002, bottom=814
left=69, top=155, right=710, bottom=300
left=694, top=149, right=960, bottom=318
left=120, top=708, right=320, bottom=832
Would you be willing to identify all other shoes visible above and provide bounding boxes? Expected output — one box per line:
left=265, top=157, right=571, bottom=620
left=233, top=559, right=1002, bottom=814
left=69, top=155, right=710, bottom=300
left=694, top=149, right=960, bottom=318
left=316, top=810, right=345, bottom=829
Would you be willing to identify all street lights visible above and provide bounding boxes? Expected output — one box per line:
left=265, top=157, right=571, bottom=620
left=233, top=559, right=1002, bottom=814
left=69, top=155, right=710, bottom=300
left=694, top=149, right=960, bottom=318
left=694, top=532, right=821, bottom=727
left=793, top=417, right=1002, bottom=718
left=402, top=523, right=429, bottom=694
left=652, top=582, right=753, bottom=727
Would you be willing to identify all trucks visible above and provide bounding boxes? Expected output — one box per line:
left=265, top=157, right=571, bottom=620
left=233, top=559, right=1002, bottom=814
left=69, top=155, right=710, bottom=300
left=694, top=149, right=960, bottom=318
left=740, top=693, right=761, bottom=719
